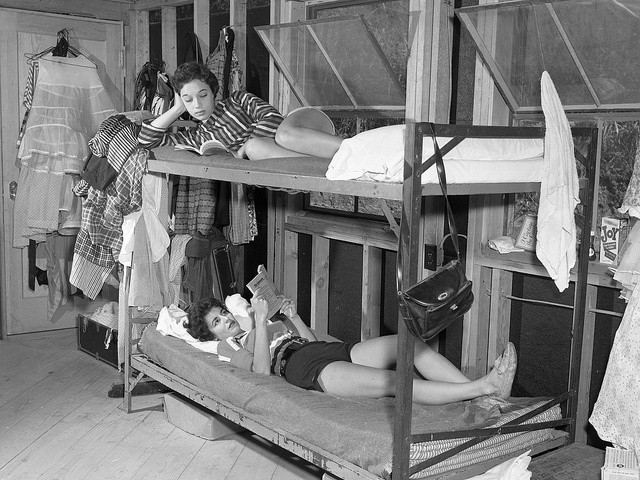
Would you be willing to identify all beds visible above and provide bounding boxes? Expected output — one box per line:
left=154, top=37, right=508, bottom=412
left=120, top=105, right=600, bottom=480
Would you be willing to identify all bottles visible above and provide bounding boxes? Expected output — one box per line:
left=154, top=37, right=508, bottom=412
left=588, top=231, right=596, bottom=261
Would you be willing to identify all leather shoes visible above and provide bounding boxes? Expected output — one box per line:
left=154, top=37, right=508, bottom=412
left=495, top=342, right=518, bottom=400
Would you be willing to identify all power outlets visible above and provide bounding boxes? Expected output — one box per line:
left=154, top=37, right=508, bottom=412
left=424, top=243, right=437, bottom=271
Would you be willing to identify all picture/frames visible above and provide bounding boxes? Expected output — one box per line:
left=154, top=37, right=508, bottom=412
left=514, top=215, right=538, bottom=253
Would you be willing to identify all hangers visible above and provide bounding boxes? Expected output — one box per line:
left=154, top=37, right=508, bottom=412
left=24, top=29, right=101, bottom=71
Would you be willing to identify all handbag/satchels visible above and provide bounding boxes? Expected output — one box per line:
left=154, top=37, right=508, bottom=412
left=397, top=259, right=475, bottom=344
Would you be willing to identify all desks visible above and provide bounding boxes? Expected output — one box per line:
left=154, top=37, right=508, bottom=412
left=527, top=441, right=605, bottom=480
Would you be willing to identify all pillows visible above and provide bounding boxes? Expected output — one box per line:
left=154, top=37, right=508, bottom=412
left=323, top=121, right=547, bottom=183
left=185, top=293, right=272, bottom=357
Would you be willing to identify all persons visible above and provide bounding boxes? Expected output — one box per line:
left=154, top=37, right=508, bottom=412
left=137, top=63, right=344, bottom=162
left=182, top=295, right=519, bottom=405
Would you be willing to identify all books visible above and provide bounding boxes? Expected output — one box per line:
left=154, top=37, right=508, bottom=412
left=246, top=264, right=284, bottom=321
left=175, top=140, right=226, bottom=156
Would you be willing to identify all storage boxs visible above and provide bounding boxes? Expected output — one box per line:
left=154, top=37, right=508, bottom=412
left=77, top=312, right=123, bottom=369
